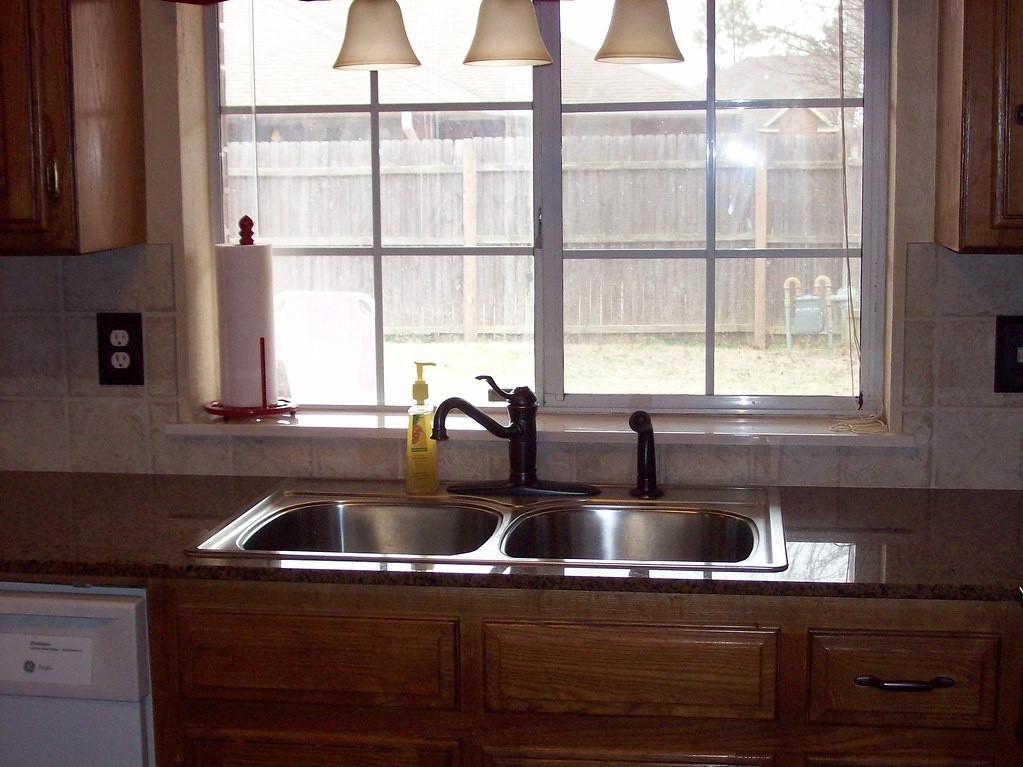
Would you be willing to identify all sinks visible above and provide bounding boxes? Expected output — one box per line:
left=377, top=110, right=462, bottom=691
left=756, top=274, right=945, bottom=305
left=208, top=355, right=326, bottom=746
left=183, top=487, right=504, bottom=563
left=499, top=486, right=787, bottom=573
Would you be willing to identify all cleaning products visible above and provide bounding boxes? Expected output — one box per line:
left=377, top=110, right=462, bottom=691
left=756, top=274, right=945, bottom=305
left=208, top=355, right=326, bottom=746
left=405, top=362, right=439, bottom=496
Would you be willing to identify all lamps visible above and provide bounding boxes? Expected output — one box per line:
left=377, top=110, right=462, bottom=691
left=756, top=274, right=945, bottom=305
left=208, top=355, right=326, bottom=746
left=332, top=0, right=422, bottom=71
left=594, top=0, right=685, bottom=64
left=462, top=0, right=553, bottom=67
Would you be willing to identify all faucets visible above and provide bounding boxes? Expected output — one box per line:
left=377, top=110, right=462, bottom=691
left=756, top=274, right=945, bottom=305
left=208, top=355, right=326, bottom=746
left=429, top=375, right=540, bottom=482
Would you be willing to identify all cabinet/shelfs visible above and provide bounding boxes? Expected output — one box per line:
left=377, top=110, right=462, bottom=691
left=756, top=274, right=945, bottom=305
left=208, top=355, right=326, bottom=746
left=166, top=580, right=1023, bottom=767
left=934, top=0, right=1023, bottom=254
left=0, top=0, right=147, bottom=255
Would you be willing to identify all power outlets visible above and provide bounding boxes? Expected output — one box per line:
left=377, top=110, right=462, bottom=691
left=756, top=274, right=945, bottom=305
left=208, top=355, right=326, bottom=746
left=96, top=312, right=144, bottom=386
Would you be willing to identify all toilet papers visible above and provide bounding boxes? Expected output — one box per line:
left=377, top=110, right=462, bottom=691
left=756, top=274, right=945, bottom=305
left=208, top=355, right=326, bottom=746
left=215, top=242, right=278, bottom=408
